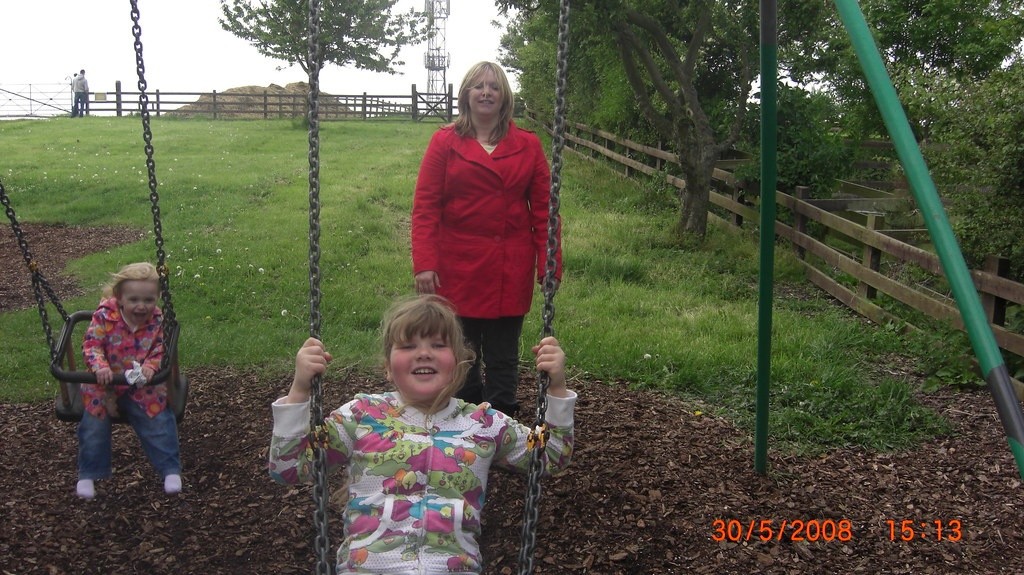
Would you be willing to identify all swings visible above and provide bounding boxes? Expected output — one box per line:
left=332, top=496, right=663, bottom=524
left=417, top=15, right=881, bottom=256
left=0, top=1, right=193, bottom=428
left=298, top=0, right=575, bottom=575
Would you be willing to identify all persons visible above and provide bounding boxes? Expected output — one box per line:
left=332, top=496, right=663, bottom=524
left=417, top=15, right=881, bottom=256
left=411, top=62, right=562, bottom=423
left=72, top=70, right=89, bottom=118
left=271, top=294, right=577, bottom=575
left=77, top=262, right=182, bottom=497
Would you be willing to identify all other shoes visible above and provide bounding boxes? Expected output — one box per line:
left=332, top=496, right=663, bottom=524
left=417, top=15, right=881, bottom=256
left=164, top=473, right=182, bottom=493
left=77, top=479, right=95, bottom=499
